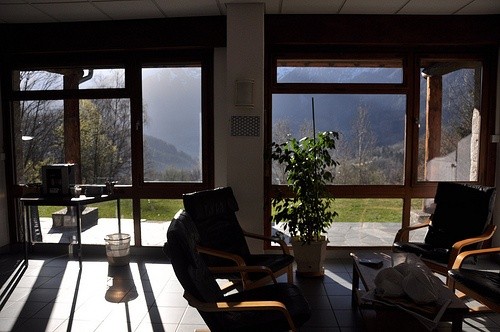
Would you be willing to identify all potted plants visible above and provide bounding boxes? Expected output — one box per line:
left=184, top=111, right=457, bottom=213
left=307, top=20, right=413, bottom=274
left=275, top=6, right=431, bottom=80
left=263, top=130, right=339, bottom=278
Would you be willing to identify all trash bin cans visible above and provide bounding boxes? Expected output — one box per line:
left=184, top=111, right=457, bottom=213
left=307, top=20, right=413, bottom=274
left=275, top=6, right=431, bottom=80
left=103, top=233, right=130, bottom=267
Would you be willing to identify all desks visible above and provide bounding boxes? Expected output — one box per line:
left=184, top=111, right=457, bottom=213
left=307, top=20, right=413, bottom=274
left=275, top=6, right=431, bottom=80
left=348, top=252, right=496, bottom=332
left=19, top=194, right=121, bottom=271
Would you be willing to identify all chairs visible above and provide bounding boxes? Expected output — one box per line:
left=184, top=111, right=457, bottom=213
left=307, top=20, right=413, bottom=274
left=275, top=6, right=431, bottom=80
left=162, top=208, right=314, bottom=332
left=394, top=182, right=497, bottom=285
left=447, top=247, right=500, bottom=313
left=182, top=186, right=295, bottom=290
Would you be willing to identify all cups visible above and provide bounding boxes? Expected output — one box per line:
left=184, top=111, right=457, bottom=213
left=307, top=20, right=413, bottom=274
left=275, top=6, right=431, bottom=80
left=70, top=188, right=82, bottom=198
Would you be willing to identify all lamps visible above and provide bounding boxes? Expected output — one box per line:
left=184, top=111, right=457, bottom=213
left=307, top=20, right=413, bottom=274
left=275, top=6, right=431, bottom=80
left=233, top=78, right=256, bottom=109
